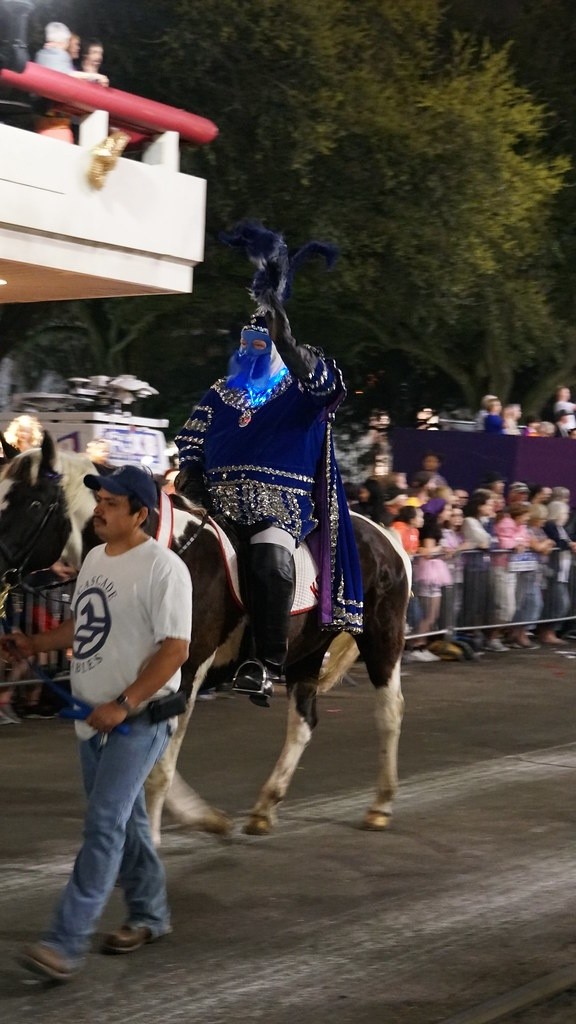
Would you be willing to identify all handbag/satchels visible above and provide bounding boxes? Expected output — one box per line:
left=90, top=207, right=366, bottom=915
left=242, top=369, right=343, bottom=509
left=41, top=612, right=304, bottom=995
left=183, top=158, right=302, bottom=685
left=507, top=552, right=539, bottom=571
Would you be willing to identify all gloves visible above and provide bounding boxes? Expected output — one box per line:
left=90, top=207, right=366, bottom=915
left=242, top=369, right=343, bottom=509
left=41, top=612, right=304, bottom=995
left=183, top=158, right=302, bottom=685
left=174, top=461, right=211, bottom=507
left=265, top=290, right=317, bottom=378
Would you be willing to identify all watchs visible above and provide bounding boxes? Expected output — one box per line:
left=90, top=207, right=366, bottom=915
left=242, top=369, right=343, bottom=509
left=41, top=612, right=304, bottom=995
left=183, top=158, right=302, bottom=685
left=117, top=695, right=130, bottom=711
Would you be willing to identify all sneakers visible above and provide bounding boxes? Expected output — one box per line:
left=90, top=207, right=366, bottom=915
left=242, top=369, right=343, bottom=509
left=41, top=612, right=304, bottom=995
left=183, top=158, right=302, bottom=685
left=403, top=648, right=441, bottom=662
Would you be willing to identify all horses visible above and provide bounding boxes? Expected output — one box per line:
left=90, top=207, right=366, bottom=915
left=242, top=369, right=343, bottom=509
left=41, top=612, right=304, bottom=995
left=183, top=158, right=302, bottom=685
left=0, top=428, right=415, bottom=837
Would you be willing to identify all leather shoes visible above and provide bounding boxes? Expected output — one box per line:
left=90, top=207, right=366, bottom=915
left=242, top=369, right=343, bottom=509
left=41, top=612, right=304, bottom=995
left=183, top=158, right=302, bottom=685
left=16, top=932, right=86, bottom=979
left=99, top=919, right=172, bottom=950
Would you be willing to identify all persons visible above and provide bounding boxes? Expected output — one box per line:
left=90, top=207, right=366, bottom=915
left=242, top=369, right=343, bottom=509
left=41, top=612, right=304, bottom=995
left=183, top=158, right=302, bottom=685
left=85, top=439, right=110, bottom=463
left=175, top=291, right=363, bottom=708
left=3, top=415, right=42, bottom=453
left=31, top=23, right=109, bottom=144
left=478, top=386, right=576, bottom=440
left=0, top=465, right=192, bottom=981
left=0, top=561, right=76, bottom=726
left=344, top=453, right=576, bottom=664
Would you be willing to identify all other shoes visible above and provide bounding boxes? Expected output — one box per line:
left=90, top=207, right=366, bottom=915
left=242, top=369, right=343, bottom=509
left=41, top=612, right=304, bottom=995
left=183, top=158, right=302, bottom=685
left=480, top=639, right=510, bottom=652
left=236, top=671, right=271, bottom=708
left=512, top=636, right=540, bottom=649
left=541, top=638, right=570, bottom=647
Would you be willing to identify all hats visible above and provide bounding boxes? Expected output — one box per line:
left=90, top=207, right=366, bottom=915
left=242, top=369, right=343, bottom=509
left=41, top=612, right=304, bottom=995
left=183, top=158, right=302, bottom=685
left=483, top=395, right=497, bottom=404
left=508, top=481, right=530, bottom=492
left=83, top=465, right=157, bottom=516
